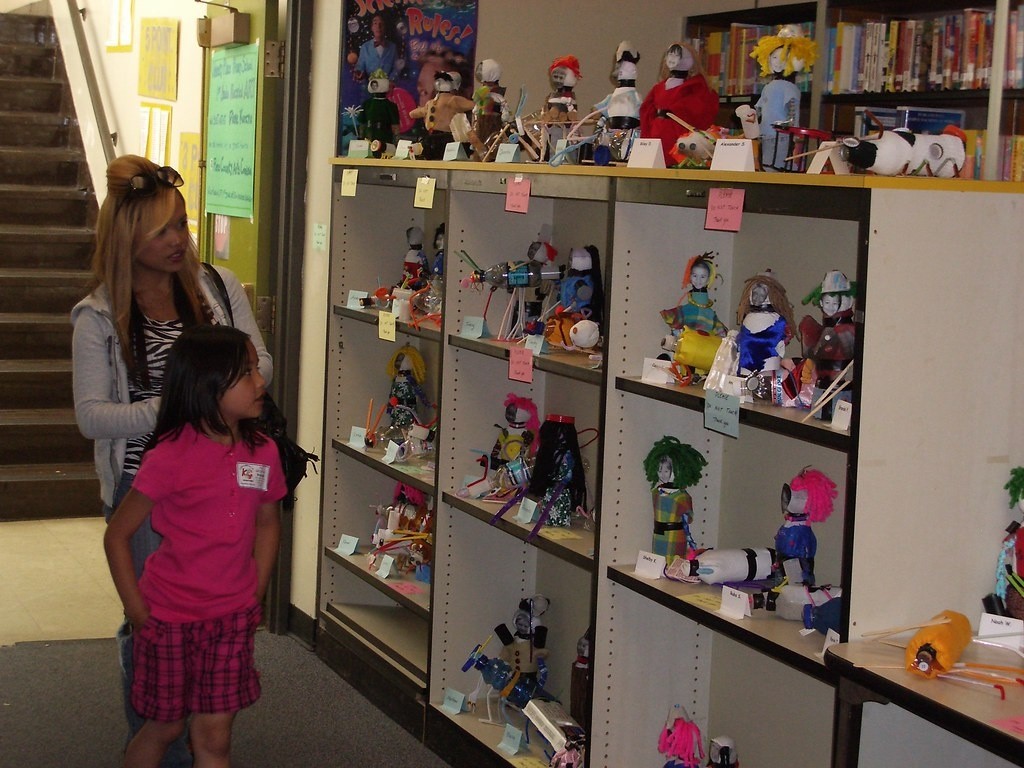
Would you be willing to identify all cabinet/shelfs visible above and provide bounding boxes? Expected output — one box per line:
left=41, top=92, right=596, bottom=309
left=679, top=0, right=1024, bottom=180
left=314, top=154, right=1024, bottom=768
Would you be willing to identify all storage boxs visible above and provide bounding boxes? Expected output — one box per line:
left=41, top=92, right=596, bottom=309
left=977, top=613, right=1024, bottom=649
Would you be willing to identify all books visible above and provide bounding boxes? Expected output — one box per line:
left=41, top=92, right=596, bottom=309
left=683, top=4, right=1024, bottom=182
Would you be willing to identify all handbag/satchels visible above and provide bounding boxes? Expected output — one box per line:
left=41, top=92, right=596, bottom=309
left=262, top=425, right=320, bottom=512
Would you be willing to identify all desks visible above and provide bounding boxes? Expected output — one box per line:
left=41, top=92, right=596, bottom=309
left=823, top=630, right=1024, bottom=768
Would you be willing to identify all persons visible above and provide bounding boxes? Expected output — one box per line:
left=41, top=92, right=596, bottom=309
left=362, top=481, right=434, bottom=583
left=470, top=59, right=512, bottom=162
left=649, top=434, right=713, bottom=584
left=658, top=704, right=737, bottom=768
left=995, top=467, right=1024, bottom=620
left=454, top=221, right=606, bottom=355
left=354, top=13, right=470, bottom=110
left=356, top=68, right=403, bottom=160
left=358, top=221, right=446, bottom=332
left=660, top=251, right=858, bottom=424
left=102, top=324, right=292, bottom=768
left=364, top=342, right=439, bottom=460
left=588, top=23, right=819, bottom=172
left=547, top=55, right=585, bottom=161
left=466, top=392, right=584, bottom=544
left=492, top=593, right=590, bottom=768
left=72, top=155, right=272, bottom=768
left=766, top=465, right=840, bottom=610
left=409, top=70, right=479, bottom=160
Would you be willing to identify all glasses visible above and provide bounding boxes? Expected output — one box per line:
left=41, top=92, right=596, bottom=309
left=113, top=166, right=184, bottom=220
